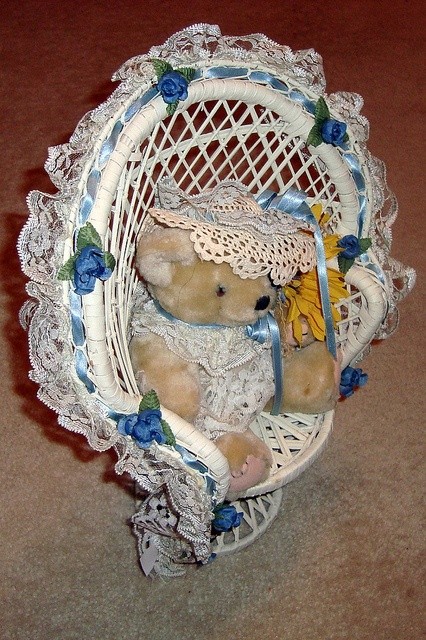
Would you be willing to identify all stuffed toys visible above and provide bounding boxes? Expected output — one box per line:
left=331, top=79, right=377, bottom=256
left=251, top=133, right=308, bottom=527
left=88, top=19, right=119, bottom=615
left=129, top=180, right=341, bottom=502
